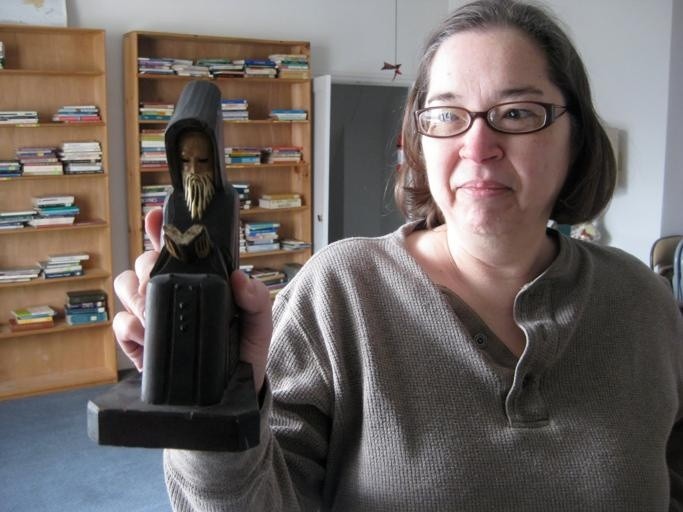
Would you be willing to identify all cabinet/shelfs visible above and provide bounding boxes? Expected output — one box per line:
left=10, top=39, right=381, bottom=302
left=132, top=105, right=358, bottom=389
left=121, top=31, right=315, bottom=309
left=0, top=23, right=119, bottom=400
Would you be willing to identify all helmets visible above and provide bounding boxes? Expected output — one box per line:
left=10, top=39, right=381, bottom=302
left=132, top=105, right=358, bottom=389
left=413, top=102, right=569, bottom=138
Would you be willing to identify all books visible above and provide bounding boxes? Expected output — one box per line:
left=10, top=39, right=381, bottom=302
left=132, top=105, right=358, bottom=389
left=0, top=104, right=109, bottom=328
left=138, top=55, right=307, bottom=290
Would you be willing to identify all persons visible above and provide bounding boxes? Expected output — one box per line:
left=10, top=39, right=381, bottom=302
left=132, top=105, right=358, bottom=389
left=110, top=0, right=682, bottom=510
left=149, top=81, right=240, bottom=385
left=135, top=131, right=241, bottom=407
